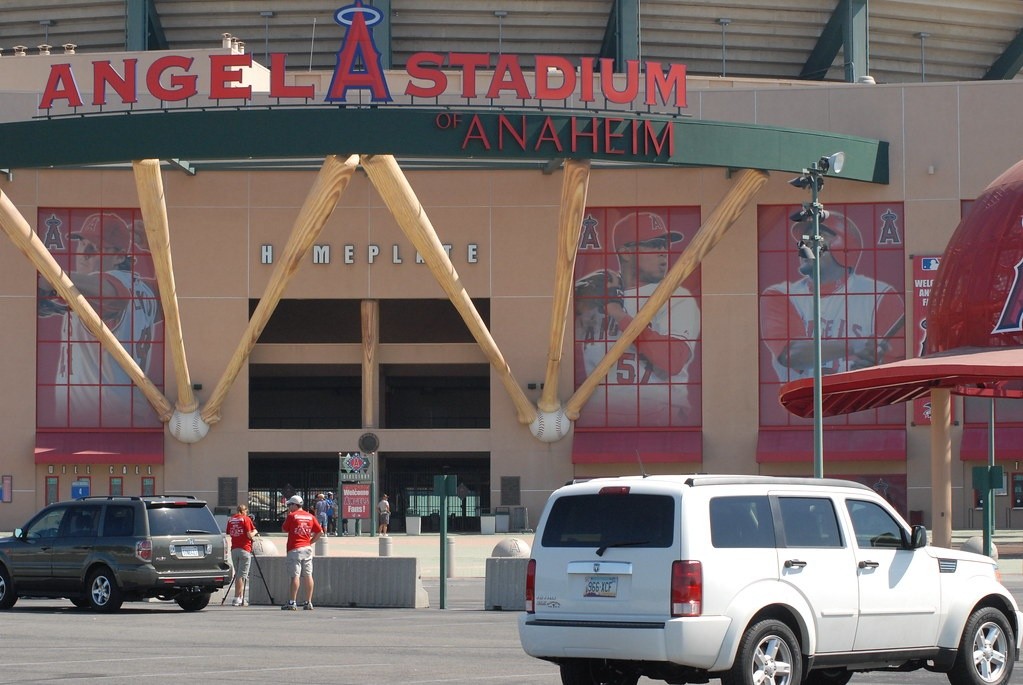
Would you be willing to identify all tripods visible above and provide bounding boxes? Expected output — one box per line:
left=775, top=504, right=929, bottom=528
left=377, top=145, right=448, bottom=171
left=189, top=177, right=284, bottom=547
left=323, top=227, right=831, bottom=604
left=221, top=540, right=275, bottom=606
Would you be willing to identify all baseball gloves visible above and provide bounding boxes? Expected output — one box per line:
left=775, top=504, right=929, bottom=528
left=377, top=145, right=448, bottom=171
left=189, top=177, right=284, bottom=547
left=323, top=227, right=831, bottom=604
left=37, top=286, right=68, bottom=318
left=575, top=268, right=625, bottom=314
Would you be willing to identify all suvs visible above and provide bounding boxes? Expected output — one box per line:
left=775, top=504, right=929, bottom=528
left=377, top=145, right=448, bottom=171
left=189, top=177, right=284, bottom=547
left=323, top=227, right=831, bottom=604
left=516, top=472, right=1023, bottom=685
left=0, top=494, right=233, bottom=614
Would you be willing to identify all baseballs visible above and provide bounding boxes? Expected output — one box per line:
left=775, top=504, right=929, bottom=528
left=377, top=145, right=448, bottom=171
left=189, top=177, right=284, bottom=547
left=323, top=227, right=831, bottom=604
left=530, top=406, right=571, bottom=443
left=126, top=223, right=141, bottom=243
left=168, top=408, right=210, bottom=444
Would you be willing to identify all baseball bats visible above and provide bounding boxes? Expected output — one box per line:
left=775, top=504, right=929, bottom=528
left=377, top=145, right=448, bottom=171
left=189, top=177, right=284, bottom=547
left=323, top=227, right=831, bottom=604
left=131, top=159, right=199, bottom=413
left=563, top=168, right=771, bottom=421
left=538, top=157, right=591, bottom=413
left=881, top=312, right=905, bottom=343
left=0, top=188, right=175, bottom=423
left=200, top=154, right=359, bottom=425
left=360, top=154, right=537, bottom=424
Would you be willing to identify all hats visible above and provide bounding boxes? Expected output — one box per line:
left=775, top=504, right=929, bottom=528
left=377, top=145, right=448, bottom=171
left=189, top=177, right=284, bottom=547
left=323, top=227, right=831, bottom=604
left=613, top=212, right=684, bottom=251
left=287, top=495, right=303, bottom=505
left=66, top=214, right=130, bottom=253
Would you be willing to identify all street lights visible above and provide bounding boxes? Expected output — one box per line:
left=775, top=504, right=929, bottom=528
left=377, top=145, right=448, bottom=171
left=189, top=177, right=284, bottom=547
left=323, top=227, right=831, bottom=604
left=793, top=151, right=845, bottom=475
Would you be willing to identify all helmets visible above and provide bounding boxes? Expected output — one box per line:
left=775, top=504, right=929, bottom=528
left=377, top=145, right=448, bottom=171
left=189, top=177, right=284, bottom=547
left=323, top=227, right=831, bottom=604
left=791, top=209, right=863, bottom=268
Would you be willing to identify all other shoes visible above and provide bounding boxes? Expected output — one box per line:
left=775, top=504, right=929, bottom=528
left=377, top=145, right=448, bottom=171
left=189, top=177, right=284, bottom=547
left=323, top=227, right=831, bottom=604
left=379, top=534, right=382, bottom=536
left=232, top=599, right=248, bottom=606
left=384, top=534, right=388, bottom=536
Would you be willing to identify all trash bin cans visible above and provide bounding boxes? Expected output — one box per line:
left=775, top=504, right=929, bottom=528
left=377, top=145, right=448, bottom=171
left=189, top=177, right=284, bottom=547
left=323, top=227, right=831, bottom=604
left=513, top=506, right=529, bottom=533
left=480, top=507, right=495, bottom=535
left=496, top=506, right=510, bottom=533
left=405, top=509, right=422, bottom=536
left=213, top=507, right=231, bottom=533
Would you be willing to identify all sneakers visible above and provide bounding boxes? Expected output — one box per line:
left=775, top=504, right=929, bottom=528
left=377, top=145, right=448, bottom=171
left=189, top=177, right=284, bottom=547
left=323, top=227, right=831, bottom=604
left=303, top=601, right=313, bottom=610
left=281, top=601, right=297, bottom=610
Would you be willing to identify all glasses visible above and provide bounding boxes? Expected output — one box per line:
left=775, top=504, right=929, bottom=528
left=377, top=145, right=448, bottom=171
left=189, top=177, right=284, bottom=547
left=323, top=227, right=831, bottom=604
left=624, top=238, right=672, bottom=250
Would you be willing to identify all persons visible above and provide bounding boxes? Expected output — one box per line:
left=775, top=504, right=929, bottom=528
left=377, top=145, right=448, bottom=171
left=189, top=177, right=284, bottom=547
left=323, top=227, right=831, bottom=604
left=281, top=495, right=324, bottom=610
left=225, top=504, right=257, bottom=606
left=310, top=492, right=348, bottom=536
left=576, top=212, right=700, bottom=427
left=376, top=494, right=390, bottom=537
left=760, top=211, right=907, bottom=425
left=39, top=213, right=159, bottom=427
